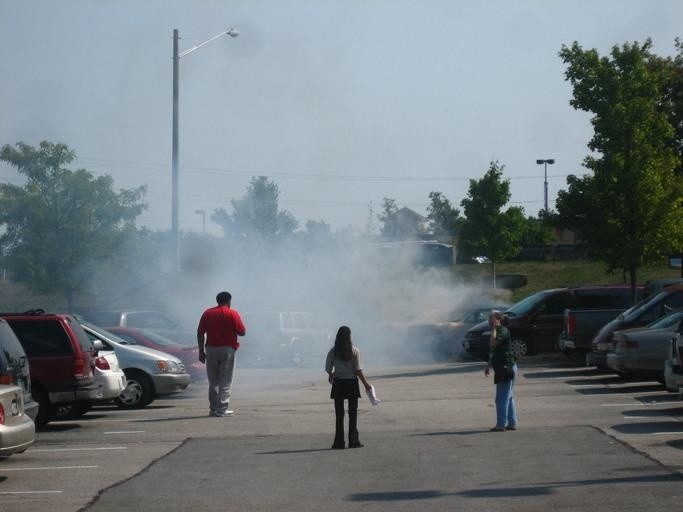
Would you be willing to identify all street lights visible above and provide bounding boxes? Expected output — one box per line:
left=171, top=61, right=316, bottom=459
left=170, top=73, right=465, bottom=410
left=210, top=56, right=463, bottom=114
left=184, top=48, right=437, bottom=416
left=535, top=157, right=555, bottom=213
left=190, top=204, right=210, bottom=236
left=165, top=25, right=242, bottom=299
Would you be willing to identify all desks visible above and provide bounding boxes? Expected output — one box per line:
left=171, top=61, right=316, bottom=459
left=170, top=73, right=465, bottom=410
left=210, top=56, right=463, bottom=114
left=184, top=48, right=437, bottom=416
left=535, top=157, right=555, bottom=213
left=165, top=25, right=242, bottom=299
left=190, top=204, right=210, bottom=236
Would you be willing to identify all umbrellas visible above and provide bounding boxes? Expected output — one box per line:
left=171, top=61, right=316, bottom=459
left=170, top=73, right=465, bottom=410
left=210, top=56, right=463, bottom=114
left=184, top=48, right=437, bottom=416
left=489, top=425, right=517, bottom=431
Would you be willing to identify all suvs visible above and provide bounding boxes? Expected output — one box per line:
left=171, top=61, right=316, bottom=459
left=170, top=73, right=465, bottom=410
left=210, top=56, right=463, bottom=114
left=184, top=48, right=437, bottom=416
left=65, top=321, right=127, bottom=416
left=0, top=307, right=105, bottom=429
left=0, top=317, right=40, bottom=424
left=70, top=313, right=191, bottom=408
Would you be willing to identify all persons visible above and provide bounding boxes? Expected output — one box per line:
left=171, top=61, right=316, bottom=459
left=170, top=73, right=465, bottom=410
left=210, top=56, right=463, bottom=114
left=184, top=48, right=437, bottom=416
left=484, top=308, right=519, bottom=430
left=197, top=291, right=244, bottom=418
left=325, top=325, right=380, bottom=449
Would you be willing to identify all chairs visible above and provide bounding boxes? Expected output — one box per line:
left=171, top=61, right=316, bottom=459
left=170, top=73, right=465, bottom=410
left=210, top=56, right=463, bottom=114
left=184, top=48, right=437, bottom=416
left=209, top=409, right=235, bottom=417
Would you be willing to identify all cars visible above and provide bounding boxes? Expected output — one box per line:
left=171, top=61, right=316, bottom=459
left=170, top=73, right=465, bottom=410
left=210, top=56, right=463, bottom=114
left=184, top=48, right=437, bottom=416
left=372, top=281, right=682, bottom=396
left=0, top=386, right=36, bottom=464
left=98, top=326, right=208, bottom=385
